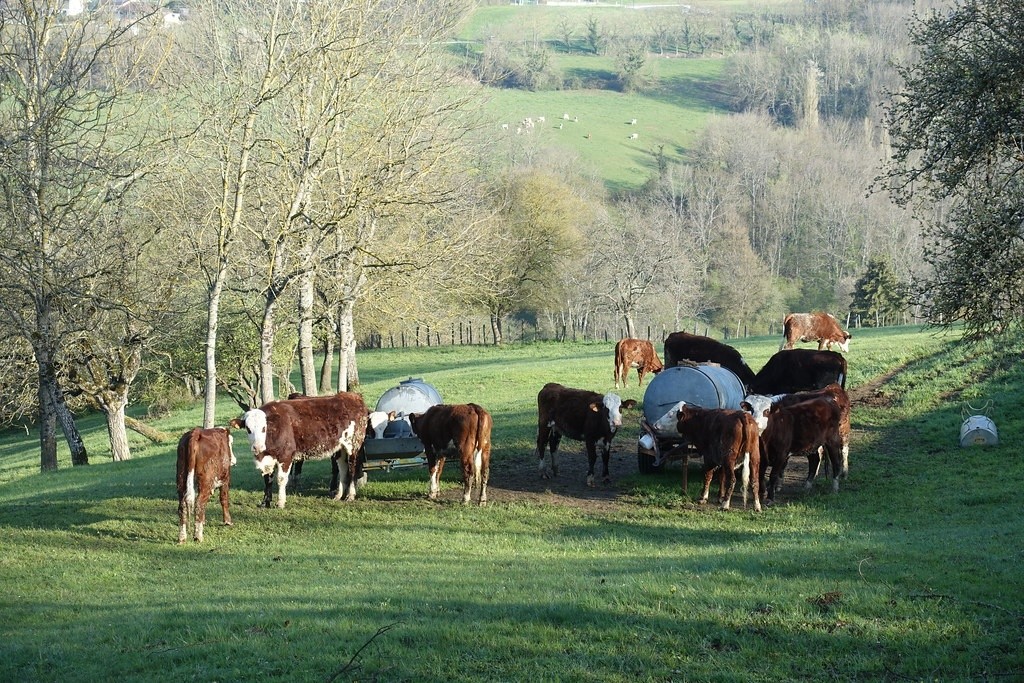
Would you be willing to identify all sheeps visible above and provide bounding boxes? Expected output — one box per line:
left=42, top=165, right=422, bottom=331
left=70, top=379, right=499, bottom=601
left=502, top=114, right=638, bottom=140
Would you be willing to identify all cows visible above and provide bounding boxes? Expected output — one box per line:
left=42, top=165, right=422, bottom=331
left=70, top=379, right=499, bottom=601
left=409, top=403, right=493, bottom=508
left=654, top=401, right=761, bottom=512
left=739, top=348, right=850, bottom=507
left=176, top=426, right=236, bottom=545
left=228, top=391, right=396, bottom=510
left=535, top=383, right=637, bottom=487
left=614, top=331, right=755, bottom=390
left=779, top=312, right=852, bottom=354
